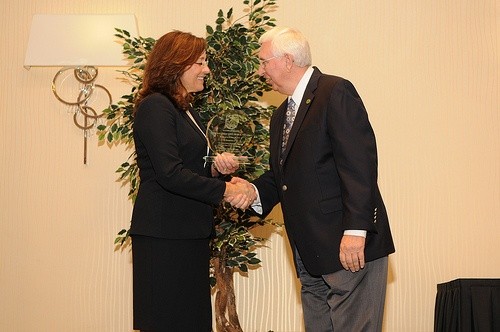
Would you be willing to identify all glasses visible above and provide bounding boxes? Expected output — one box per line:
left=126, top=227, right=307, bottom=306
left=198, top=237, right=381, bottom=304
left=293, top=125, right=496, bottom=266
left=257, top=56, right=276, bottom=68
left=196, top=60, right=209, bottom=67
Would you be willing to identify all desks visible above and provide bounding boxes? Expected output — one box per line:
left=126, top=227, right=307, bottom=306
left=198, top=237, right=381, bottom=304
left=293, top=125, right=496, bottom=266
left=433, top=278, right=500, bottom=332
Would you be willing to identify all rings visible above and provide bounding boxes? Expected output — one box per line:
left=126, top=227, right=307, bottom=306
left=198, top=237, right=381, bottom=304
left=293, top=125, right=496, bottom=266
left=224, top=167, right=228, bottom=171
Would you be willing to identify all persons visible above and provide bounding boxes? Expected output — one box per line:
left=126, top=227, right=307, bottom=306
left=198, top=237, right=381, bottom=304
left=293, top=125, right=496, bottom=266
left=223, top=24, right=395, bottom=332
left=129, top=31, right=257, bottom=332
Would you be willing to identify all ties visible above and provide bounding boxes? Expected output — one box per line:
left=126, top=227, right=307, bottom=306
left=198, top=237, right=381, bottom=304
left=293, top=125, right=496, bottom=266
left=281, top=101, right=296, bottom=153
